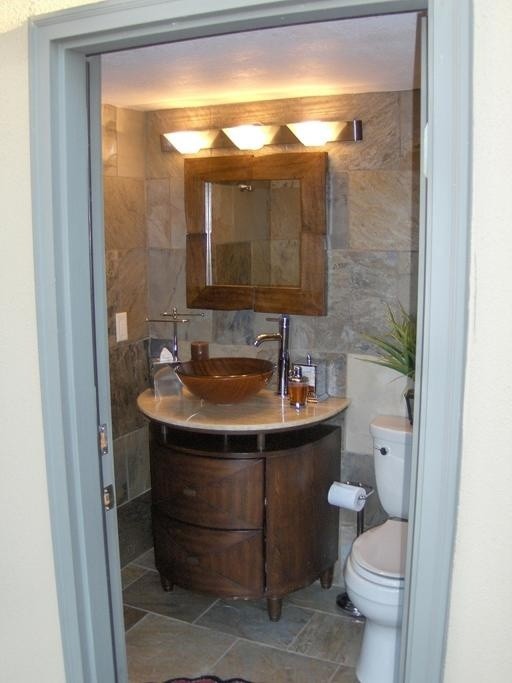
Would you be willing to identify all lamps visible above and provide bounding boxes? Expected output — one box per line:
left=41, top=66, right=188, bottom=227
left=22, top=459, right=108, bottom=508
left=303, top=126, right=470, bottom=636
left=160, top=120, right=347, bottom=157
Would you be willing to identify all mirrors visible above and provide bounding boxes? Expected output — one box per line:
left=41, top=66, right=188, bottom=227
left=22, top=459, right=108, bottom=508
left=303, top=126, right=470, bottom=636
left=182, top=153, right=327, bottom=316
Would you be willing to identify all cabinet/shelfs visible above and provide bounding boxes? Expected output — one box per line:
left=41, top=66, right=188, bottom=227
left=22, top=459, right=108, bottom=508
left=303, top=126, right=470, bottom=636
left=145, top=425, right=343, bottom=623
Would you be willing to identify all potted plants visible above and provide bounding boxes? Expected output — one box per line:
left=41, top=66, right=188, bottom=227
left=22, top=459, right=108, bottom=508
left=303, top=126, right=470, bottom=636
left=352, top=296, right=413, bottom=428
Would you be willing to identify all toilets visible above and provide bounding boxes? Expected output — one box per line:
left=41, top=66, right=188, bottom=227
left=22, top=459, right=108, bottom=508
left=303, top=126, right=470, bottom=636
left=343, top=414, right=413, bottom=683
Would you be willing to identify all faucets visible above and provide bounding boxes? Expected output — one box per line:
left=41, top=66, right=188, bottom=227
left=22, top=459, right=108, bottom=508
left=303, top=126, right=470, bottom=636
left=253, top=315, right=290, bottom=395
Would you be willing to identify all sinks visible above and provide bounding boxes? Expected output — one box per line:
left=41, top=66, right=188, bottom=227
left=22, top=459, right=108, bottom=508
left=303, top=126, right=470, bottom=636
left=174, top=358, right=277, bottom=404
